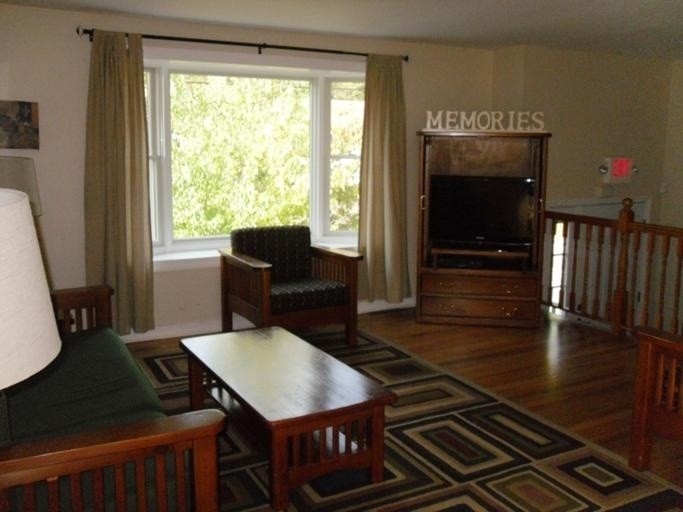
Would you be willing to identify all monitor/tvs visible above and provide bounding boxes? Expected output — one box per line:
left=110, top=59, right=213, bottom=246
left=428, top=174, right=536, bottom=252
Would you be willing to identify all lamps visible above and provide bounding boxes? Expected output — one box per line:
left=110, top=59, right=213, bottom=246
left=1, top=187, right=63, bottom=392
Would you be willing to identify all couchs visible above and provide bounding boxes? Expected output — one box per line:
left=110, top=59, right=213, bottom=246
left=1, top=285, right=228, bottom=510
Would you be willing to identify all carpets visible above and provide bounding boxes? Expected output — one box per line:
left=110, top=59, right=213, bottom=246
left=123, top=322, right=683, bottom=511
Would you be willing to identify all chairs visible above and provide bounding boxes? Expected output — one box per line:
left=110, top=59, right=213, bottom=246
left=218, top=225, right=364, bottom=345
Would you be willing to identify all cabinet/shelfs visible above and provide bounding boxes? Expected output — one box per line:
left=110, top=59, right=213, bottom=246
left=414, top=130, right=551, bottom=329
